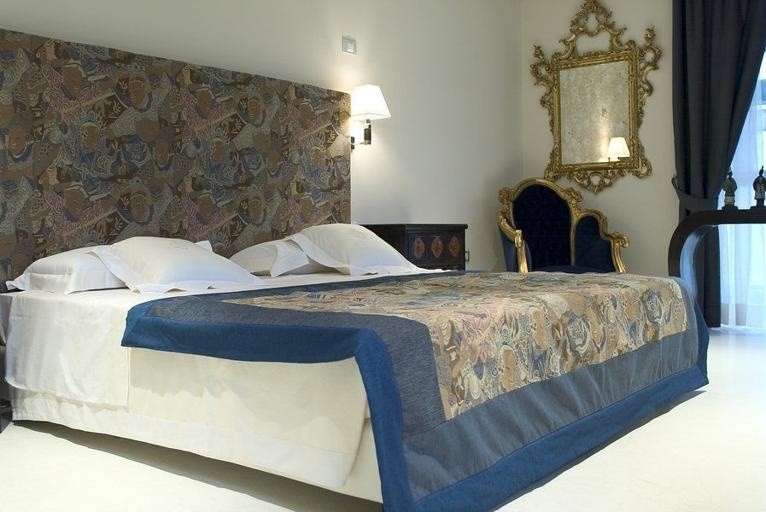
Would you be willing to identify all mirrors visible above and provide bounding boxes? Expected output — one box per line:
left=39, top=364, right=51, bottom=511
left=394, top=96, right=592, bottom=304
left=526, top=1, right=664, bottom=197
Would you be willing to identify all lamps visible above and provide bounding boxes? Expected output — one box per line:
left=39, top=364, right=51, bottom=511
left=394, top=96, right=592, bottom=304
left=349, top=82, right=392, bottom=153
left=604, top=136, right=631, bottom=164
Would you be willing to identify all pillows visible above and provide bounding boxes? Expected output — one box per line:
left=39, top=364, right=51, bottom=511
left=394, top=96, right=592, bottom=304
left=5, top=240, right=125, bottom=296
left=281, top=220, right=444, bottom=277
left=227, top=236, right=337, bottom=279
left=87, top=237, right=270, bottom=298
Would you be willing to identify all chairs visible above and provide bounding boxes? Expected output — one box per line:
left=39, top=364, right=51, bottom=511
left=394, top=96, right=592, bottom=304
left=495, top=175, right=629, bottom=276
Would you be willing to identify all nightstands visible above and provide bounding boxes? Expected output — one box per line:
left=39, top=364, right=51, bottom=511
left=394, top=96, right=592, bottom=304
left=360, top=220, right=470, bottom=273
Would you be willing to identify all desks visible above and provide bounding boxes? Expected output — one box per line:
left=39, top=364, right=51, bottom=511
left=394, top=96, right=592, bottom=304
left=665, top=204, right=766, bottom=331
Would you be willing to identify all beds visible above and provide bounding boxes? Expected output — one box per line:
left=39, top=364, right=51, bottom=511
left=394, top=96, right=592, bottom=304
left=0, top=24, right=714, bottom=512
